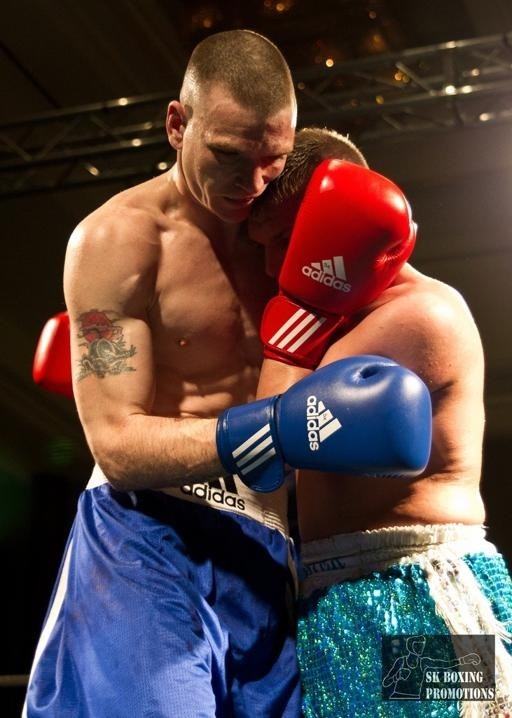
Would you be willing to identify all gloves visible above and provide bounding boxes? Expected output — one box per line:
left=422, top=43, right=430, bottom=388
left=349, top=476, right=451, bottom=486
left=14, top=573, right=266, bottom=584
left=32, top=311, right=74, bottom=399
left=215, top=355, right=433, bottom=494
left=258, top=157, right=418, bottom=370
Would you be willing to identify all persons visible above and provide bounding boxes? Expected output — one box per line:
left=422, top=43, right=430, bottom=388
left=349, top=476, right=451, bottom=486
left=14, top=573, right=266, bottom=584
left=21, top=30, right=432, bottom=718
left=32, top=127, right=512, bottom=718
